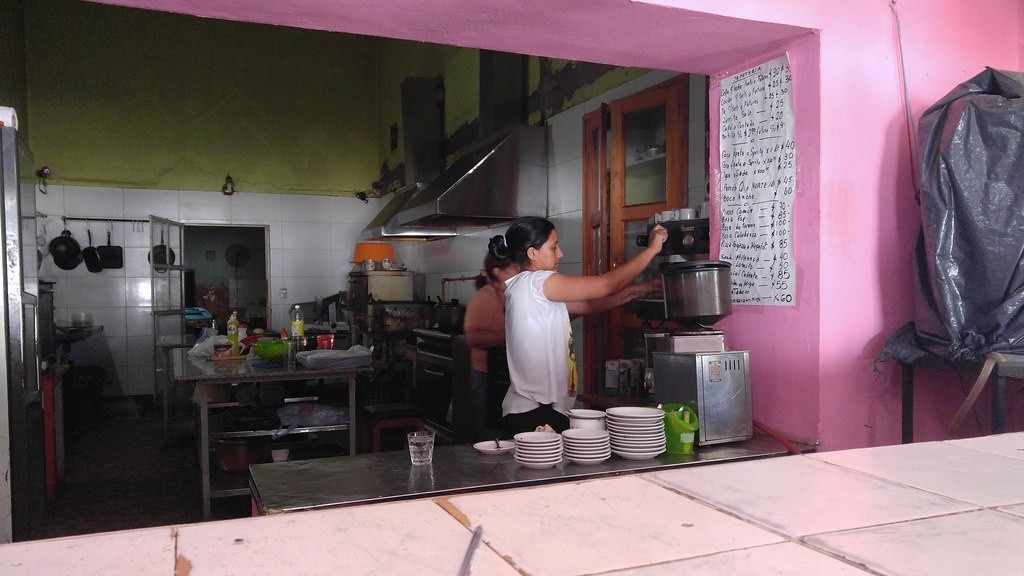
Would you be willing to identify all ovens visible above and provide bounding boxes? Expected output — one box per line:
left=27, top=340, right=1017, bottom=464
left=413, top=359, right=456, bottom=444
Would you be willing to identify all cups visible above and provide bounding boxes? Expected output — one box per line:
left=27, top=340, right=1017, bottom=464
left=283, top=341, right=296, bottom=366
left=648, top=202, right=709, bottom=224
left=408, top=431, right=435, bottom=465
left=317, top=334, right=334, bottom=350
left=568, top=409, right=607, bottom=431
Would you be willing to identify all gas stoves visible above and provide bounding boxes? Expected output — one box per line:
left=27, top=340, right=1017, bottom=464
left=412, top=328, right=468, bottom=368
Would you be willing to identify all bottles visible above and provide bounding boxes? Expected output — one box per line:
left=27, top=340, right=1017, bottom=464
left=290, top=305, right=304, bottom=352
left=227, top=314, right=239, bottom=356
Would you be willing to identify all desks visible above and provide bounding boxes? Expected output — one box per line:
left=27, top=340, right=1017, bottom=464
left=249, top=431, right=816, bottom=518
left=576, top=390, right=654, bottom=409
left=172, top=348, right=372, bottom=521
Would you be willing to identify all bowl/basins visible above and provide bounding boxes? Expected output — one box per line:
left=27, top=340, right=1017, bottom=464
left=250, top=339, right=291, bottom=361
left=291, top=335, right=317, bottom=354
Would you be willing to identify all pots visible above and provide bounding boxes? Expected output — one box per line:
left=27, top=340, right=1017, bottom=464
left=50, top=220, right=83, bottom=269
left=437, top=296, right=466, bottom=332
left=82, top=229, right=103, bottom=273
left=148, top=230, right=175, bottom=273
left=97, top=232, right=123, bottom=268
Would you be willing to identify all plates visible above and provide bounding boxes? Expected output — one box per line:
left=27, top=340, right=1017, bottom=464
left=513, top=429, right=611, bottom=469
left=605, top=407, right=666, bottom=460
left=473, top=440, right=515, bottom=455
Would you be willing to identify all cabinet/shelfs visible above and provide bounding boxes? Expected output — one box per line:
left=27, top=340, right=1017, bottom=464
left=580, top=71, right=687, bottom=409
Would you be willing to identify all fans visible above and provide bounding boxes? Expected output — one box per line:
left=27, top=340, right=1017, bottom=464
left=226, top=244, right=250, bottom=268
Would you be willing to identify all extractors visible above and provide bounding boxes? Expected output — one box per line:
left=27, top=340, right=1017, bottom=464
left=360, top=47, right=547, bottom=241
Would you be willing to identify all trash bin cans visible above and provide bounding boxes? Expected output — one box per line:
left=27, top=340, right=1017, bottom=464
left=62, top=366, right=108, bottom=433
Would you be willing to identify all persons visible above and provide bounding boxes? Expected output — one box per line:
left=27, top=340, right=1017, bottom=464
left=463, top=247, right=583, bottom=441
left=488, top=217, right=668, bottom=440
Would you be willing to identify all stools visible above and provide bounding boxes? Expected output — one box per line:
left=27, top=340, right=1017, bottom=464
left=359, top=402, right=423, bottom=453
